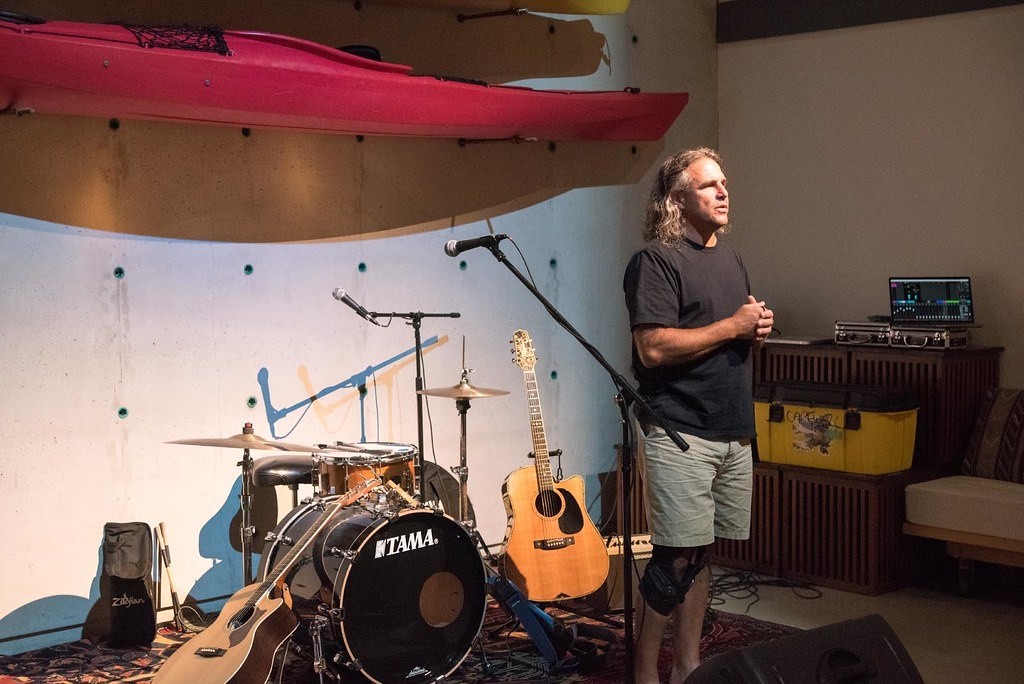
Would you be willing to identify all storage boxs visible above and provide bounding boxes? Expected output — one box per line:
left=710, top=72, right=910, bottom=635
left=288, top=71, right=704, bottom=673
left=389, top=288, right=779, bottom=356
left=834, top=321, right=968, bottom=350
left=753, top=379, right=921, bottom=476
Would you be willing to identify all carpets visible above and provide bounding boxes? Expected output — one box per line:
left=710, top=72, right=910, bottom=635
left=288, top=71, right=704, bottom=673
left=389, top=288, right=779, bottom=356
left=0, top=553, right=809, bottom=684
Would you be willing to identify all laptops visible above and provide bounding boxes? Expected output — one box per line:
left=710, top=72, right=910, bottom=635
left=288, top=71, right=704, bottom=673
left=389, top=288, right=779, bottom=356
left=888, top=277, right=983, bottom=329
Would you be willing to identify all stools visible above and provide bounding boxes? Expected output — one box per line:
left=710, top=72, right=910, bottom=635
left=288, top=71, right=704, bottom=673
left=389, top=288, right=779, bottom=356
left=252, top=456, right=319, bottom=510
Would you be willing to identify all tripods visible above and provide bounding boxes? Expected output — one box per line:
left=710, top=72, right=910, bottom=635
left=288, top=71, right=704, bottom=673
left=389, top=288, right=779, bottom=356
left=487, top=449, right=624, bottom=637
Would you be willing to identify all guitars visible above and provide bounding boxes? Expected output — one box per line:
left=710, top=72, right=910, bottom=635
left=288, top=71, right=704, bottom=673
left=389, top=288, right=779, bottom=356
left=150, top=463, right=383, bottom=684
left=497, top=328, right=611, bottom=604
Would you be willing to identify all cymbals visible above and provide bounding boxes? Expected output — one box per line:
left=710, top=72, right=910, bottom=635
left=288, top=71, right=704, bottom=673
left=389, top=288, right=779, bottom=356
left=161, top=434, right=322, bottom=453
left=416, top=383, right=511, bottom=401
left=311, top=441, right=417, bottom=497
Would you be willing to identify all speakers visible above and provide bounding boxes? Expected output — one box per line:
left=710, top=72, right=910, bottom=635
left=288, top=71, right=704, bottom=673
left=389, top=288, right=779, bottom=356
left=683, top=614, right=925, bottom=683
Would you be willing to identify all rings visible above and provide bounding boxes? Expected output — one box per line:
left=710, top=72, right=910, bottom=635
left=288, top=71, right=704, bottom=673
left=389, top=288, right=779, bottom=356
left=760, top=306, right=765, bottom=311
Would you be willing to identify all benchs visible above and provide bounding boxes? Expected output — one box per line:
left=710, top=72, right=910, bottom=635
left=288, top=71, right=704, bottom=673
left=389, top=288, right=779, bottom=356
left=903, top=387, right=1024, bottom=598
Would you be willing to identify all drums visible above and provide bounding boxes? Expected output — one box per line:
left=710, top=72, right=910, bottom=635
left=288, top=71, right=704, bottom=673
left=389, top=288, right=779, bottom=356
left=256, top=495, right=489, bottom=684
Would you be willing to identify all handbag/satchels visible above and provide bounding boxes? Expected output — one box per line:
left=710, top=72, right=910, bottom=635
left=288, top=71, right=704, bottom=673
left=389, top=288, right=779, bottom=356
left=104, top=522, right=157, bottom=644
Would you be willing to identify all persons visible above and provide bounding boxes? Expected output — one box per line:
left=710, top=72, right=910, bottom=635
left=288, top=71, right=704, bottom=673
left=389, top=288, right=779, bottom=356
left=623, top=145, right=774, bottom=684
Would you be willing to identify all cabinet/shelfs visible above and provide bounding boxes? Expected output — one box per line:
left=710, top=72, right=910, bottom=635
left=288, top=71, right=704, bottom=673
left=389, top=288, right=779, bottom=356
left=613, top=346, right=1004, bottom=597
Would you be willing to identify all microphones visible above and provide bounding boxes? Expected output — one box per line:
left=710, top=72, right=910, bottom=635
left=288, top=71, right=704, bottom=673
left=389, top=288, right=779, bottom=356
left=444, top=233, right=507, bottom=257
left=332, top=288, right=379, bottom=326
